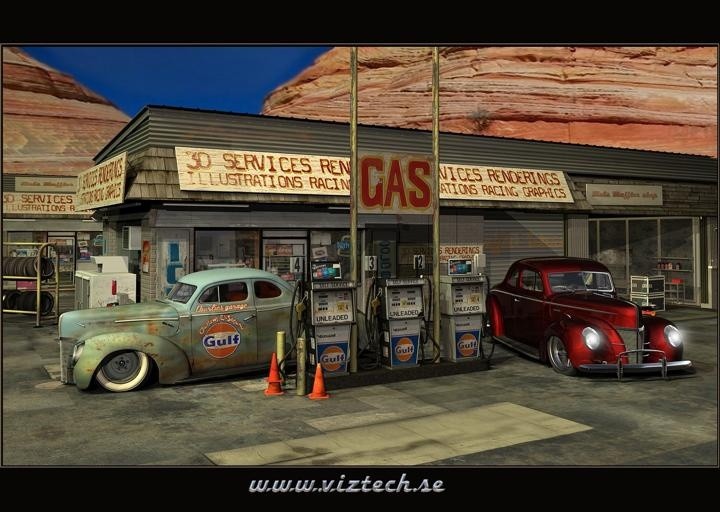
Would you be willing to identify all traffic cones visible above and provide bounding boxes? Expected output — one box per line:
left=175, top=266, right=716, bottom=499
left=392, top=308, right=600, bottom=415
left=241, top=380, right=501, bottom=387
left=309, top=362, right=331, bottom=401
left=264, top=352, right=286, bottom=396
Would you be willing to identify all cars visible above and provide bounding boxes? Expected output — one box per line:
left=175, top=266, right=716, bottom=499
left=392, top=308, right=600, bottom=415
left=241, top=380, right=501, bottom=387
left=485, top=255, right=692, bottom=381
left=58, top=267, right=302, bottom=393
